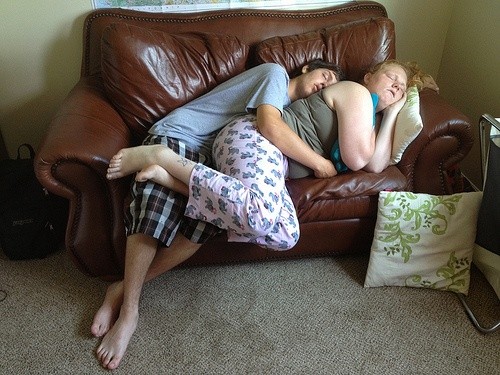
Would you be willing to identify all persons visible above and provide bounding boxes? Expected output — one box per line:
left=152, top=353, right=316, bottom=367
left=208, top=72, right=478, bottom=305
left=106, top=59, right=422, bottom=252
left=90, top=57, right=344, bottom=369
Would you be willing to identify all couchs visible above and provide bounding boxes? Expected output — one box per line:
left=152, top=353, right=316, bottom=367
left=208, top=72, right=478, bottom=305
left=31, top=0, right=477, bottom=283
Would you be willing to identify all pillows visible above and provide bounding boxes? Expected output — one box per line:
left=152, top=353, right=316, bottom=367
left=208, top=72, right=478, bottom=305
left=363, top=189, right=484, bottom=296
left=386, top=82, right=423, bottom=165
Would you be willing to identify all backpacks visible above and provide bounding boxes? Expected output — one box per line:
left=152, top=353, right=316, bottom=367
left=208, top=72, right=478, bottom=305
left=0, top=144, right=69, bottom=261
